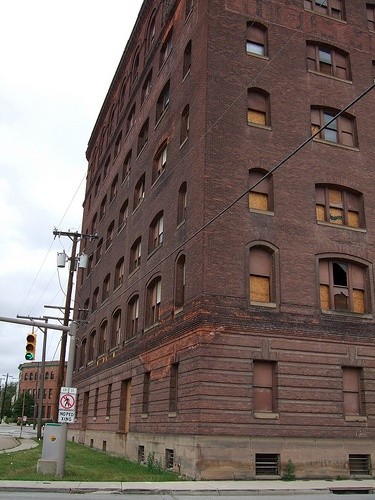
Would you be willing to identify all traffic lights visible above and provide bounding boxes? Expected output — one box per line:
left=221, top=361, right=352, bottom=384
left=25, top=334, right=36, bottom=361
